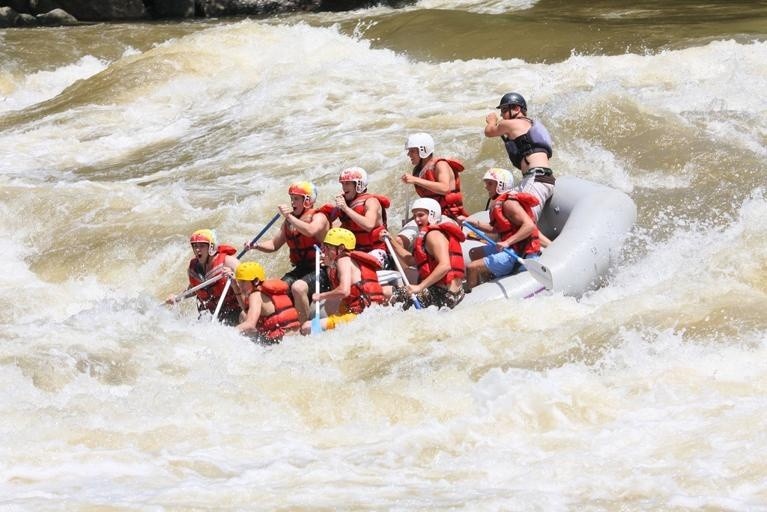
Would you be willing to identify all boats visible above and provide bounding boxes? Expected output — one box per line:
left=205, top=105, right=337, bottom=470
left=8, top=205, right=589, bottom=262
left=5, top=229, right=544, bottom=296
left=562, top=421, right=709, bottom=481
left=246, top=173, right=642, bottom=352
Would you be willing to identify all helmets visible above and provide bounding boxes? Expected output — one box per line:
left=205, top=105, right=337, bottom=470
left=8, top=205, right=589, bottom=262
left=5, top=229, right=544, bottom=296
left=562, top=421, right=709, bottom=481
left=483, top=167, right=514, bottom=195
left=232, top=261, right=266, bottom=283
left=339, top=167, right=369, bottom=193
left=412, top=198, right=443, bottom=224
left=289, top=180, right=319, bottom=208
left=496, top=93, right=528, bottom=111
left=324, top=227, right=357, bottom=251
left=405, top=133, right=436, bottom=159
left=190, top=229, right=219, bottom=257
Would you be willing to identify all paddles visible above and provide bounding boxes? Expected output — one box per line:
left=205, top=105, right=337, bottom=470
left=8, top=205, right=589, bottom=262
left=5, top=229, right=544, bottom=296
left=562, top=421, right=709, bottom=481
left=311, top=245, right=323, bottom=335
left=461, top=220, right=553, bottom=291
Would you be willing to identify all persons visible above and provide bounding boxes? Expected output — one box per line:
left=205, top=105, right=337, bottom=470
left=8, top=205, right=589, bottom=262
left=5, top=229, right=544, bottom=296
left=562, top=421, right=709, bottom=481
left=301, top=228, right=385, bottom=336
left=244, top=181, right=330, bottom=325
left=379, top=197, right=466, bottom=309
left=334, top=166, right=390, bottom=270
left=484, top=93, right=556, bottom=225
left=456, top=168, right=540, bottom=292
left=395, top=132, right=469, bottom=271
left=222, top=261, right=301, bottom=346
left=165, top=228, right=241, bottom=326
